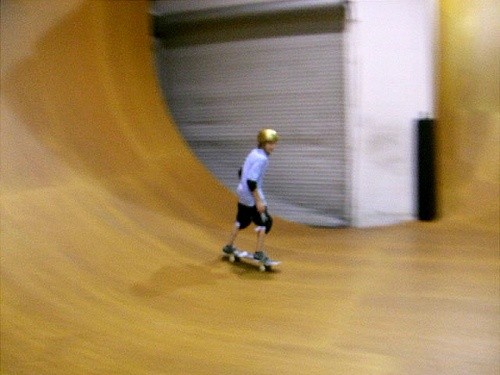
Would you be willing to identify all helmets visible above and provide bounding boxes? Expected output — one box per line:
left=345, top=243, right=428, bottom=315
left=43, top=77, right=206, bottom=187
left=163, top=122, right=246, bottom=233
left=257, top=128, right=278, bottom=142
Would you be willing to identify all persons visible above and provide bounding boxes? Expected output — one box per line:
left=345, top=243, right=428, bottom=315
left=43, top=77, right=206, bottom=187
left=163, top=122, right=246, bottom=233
left=221, top=127, right=279, bottom=263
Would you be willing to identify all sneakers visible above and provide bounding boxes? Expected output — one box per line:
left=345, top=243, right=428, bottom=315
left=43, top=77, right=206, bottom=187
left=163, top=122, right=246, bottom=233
left=223, top=245, right=236, bottom=253
left=254, top=252, right=268, bottom=261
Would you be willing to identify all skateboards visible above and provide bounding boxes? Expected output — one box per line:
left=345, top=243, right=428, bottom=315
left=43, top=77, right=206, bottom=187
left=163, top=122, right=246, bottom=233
left=223, top=249, right=280, bottom=272
